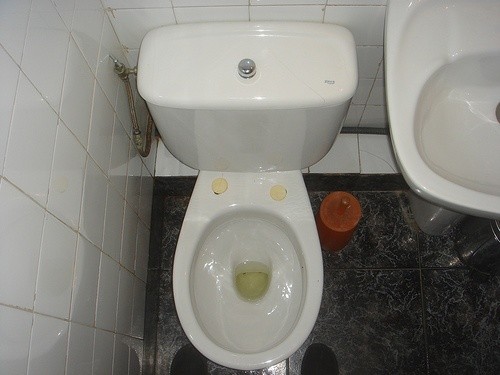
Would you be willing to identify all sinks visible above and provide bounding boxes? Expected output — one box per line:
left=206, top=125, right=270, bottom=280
left=383, top=0, right=500, bottom=221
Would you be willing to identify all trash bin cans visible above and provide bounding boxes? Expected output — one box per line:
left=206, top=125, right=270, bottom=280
left=316, top=190, right=362, bottom=251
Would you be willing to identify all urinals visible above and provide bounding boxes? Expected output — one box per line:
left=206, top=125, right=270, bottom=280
left=137, top=20, right=357, bottom=372
